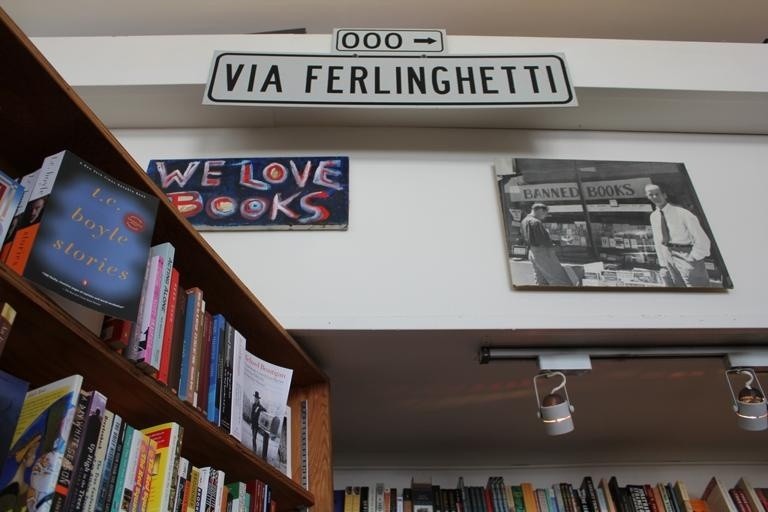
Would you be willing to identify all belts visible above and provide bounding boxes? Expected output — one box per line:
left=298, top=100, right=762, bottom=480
left=668, top=244, right=692, bottom=248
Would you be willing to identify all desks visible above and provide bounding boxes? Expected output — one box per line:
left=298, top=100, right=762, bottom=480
left=509, top=258, right=584, bottom=286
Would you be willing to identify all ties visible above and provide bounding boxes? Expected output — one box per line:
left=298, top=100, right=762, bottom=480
left=659, top=210, right=669, bottom=246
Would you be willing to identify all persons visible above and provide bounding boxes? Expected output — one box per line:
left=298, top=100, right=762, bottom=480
left=522, top=203, right=573, bottom=286
left=644, top=183, right=711, bottom=288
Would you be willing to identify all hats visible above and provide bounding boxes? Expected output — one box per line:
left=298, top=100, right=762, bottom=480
left=252, top=391, right=261, bottom=399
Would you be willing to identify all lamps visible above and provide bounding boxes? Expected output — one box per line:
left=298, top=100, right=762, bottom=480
left=478, top=348, right=768, bottom=438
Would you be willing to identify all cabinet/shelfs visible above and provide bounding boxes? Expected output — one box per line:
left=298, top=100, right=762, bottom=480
left=0, top=5, right=335, bottom=512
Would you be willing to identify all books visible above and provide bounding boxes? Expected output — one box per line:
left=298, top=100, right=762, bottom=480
left=1, top=151, right=295, bottom=511
left=332, top=476, right=767, bottom=512
